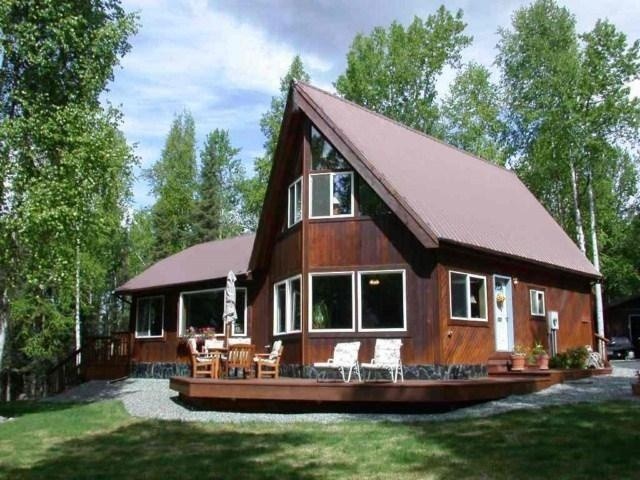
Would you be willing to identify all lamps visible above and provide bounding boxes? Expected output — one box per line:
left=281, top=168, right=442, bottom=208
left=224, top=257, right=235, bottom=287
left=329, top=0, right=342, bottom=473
left=513, top=278, right=518, bottom=284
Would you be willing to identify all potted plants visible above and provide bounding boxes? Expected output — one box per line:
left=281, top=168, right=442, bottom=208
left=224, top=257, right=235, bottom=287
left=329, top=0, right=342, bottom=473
left=509, top=341, right=527, bottom=370
left=533, top=342, right=545, bottom=364
left=631, top=371, right=640, bottom=395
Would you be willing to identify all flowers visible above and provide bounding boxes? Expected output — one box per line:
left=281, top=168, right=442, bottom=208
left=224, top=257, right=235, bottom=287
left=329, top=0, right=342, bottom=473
left=497, top=292, right=505, bottom=303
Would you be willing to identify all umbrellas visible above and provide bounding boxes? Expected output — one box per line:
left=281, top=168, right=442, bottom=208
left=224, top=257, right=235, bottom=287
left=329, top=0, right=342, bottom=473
left=220, top=270, right=239, bottom=348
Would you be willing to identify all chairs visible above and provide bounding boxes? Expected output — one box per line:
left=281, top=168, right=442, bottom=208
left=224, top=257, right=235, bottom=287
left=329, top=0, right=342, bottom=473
left=584, top=344, right=604, bottom=370
left=187, top=335, right=284, bottom=379
left=359, top=338, right=408, bottom=383
left=312, top=339, right=363, bottom=383
